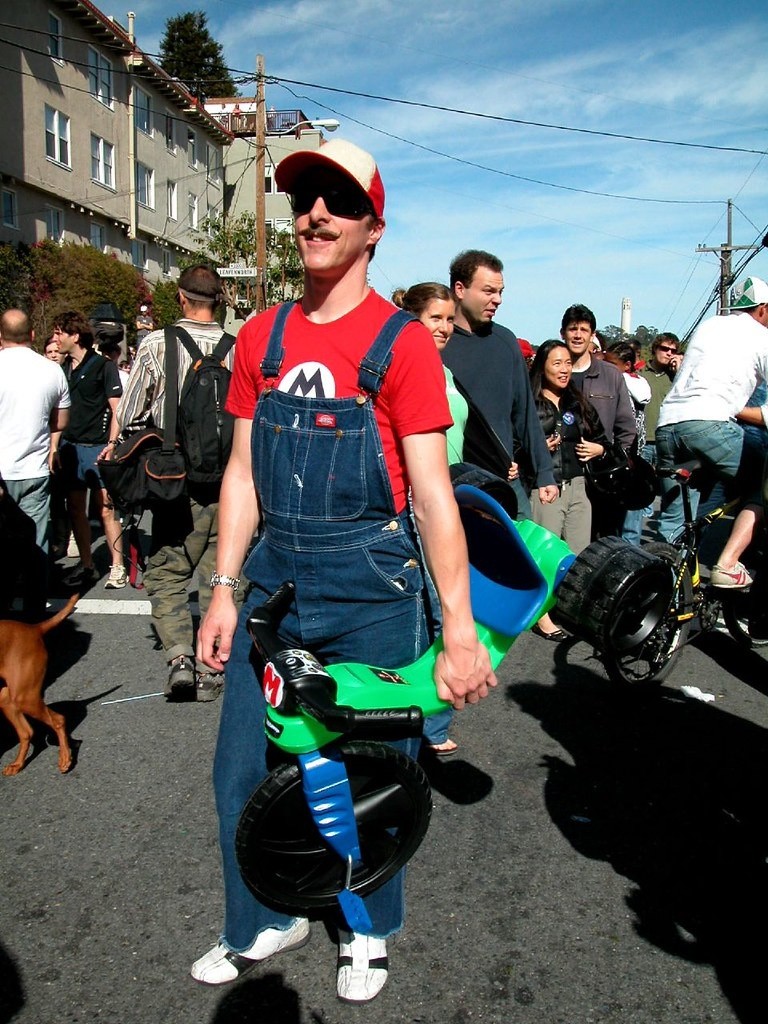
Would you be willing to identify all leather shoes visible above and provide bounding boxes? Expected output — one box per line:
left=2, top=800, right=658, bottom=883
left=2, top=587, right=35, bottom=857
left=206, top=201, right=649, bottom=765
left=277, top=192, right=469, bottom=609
left=532, top=624, right=568, bottom=641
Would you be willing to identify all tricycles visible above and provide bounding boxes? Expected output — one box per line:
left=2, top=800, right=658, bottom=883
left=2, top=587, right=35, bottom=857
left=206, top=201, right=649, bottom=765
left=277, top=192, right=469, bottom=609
left=232, top=461, right=684, bottom=937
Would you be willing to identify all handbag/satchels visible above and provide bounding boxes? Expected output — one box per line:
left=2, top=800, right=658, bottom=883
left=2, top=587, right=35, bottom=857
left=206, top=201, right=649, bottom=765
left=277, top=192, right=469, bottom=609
left=94, top=425, right=188, bottom=507
left=581, top=440, right=655, bottom=512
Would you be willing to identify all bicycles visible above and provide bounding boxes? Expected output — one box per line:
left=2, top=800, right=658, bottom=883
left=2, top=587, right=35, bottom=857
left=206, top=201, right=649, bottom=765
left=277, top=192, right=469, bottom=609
left=601, top=457, right=767, bottom=692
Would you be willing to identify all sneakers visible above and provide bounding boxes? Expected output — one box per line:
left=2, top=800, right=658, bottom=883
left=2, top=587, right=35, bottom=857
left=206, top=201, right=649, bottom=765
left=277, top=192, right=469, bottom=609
left=191, top=917, right=308, bottom=985
left=711, top=562, right=753, bottom=588
left=104, top=564, right=129, bottom=589
left=336, top=925, right=390, bottom=1000
left=63, top=563, right=100, bottom=585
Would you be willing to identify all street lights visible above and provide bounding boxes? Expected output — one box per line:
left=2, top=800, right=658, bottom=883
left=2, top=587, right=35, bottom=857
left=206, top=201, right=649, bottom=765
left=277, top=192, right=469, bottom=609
left=253, top=50, right=340, bottom=319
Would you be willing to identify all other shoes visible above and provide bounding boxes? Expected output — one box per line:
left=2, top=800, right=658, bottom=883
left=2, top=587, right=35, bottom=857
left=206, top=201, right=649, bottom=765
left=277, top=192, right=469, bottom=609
left=164, top=652, right=197, bottom=700
left=193, top=672, right=226, bottom=701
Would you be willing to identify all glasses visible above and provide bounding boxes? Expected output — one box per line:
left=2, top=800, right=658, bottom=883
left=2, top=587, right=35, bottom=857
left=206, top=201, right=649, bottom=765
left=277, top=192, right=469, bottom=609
left=289, top=182, right=377, bottom=221
left=655, top=344, right=677, bottom=354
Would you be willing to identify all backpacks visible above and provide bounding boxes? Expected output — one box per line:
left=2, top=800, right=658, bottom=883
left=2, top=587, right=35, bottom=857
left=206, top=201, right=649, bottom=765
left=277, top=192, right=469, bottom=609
left=161, top=322, right=240, bottom=508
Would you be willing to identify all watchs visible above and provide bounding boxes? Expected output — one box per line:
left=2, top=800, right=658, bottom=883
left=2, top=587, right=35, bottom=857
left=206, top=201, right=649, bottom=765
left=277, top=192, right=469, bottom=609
left=210, top=570, right=240, bottom=591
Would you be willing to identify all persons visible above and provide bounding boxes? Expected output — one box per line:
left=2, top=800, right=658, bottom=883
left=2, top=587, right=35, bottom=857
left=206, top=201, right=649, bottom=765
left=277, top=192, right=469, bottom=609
left=136, top=305, right=152, bottom=345
left=190, top=136, right=499, bottom=1000
left=52, top=310, right=129, bottom=588
left=655, top=277, right=768, bottom=588
left=115, top=265, right=235, bottom=704
left=0, top=310, right=72, bottom=605
left=437, top=249, right=559, bottom=520
left=391, top=284, right=518, bottom=755
left=516, top=304, right=686, bottom=642
left=43, top=336, right=66, bottom=364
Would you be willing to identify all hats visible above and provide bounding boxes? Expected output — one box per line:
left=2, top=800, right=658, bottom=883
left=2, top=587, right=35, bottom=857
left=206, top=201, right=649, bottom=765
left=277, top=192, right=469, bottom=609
left=730, top=277, right=768, bottom=310
left=274, top=139, right=385, bottom=221
left=517, top=339, right=536, bottom=359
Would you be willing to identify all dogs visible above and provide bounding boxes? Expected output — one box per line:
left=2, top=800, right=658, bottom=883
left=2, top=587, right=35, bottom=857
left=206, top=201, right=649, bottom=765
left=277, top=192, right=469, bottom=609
left=0, top=590, right=81, bottom=778
